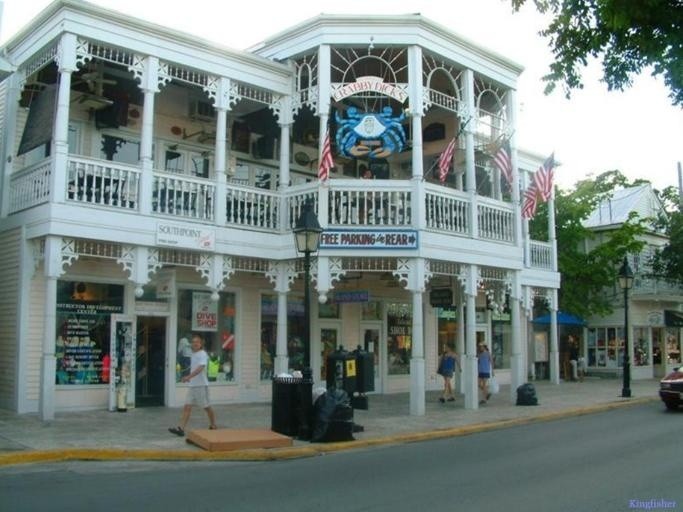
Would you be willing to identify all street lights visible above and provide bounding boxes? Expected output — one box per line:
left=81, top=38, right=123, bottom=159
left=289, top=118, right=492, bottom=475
left=290, top=196, right=325, bottom=377
left=615, top=254, right=635, bottom=398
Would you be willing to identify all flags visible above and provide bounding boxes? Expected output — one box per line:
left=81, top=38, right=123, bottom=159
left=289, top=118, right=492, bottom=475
left=520, top=179, right=538, bottom=221
left=492, top=138, right=513, bottom=194
left=533, top=152, right=555, bottom=202
left=438, top=135, right=457, bottom=186
left=317, top=126, right=334, bottom=182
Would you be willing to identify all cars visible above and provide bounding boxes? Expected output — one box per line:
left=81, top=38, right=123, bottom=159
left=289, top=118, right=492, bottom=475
left=656, top=361, right=683, bottom=411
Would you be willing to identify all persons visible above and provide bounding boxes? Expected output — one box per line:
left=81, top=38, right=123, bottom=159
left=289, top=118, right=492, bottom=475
left=567, top=339, right=588, bottom=381
left=166, top=332, right=218, bottom=436
left=476, top=340, right=496, bottom=406
left=357, top=170, right=376, bottom=223
left=435, top=341, right=462, bottom=403
left=175, top=326, right=234, bottom=383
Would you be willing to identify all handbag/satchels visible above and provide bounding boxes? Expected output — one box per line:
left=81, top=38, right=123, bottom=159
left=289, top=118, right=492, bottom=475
left=437, top=353, right=444, bottom=374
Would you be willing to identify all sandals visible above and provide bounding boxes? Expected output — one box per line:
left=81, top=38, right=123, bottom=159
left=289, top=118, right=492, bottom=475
left=167, top=427, right=183, bottom=436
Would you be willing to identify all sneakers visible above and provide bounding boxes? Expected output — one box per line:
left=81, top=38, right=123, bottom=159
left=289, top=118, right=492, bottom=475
left=479, top=400, right=486, bottom=404
left=438, top=397, right=445, bottom=403
left=447, top=398, right=455, bottom=401
left=487, top=392, right=491, bottom=400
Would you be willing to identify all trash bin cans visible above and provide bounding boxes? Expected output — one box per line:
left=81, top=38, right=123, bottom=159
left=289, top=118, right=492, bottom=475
left=272, top=377, right=313, bottom=441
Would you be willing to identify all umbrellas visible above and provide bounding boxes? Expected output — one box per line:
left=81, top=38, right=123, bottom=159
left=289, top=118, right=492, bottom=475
left=529, top=310, right=588, bottom=352
